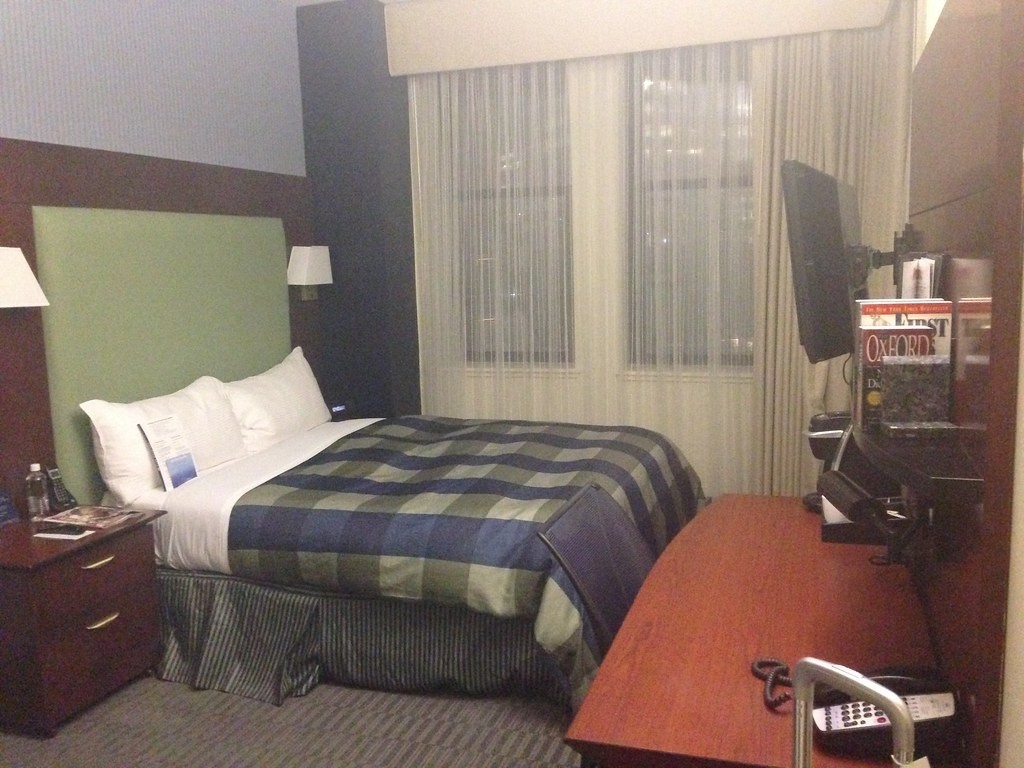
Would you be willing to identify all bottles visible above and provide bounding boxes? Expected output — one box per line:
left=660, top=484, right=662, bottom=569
left=25, top=463, right=52, bottom=534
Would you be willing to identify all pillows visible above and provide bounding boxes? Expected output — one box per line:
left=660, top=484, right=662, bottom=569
left=78, top=346, right=332, bottom=506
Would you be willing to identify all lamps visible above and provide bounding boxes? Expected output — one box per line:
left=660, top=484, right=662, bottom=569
left=0, top=247, right=51, bottom=308
left=286, top=246, right=333, bottom=301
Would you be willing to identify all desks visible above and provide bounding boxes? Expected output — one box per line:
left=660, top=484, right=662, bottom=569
left=564, top=492, right=944, bottom=768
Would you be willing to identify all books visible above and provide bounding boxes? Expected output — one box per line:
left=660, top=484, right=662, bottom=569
left=854, top=298, right=951, bottom=433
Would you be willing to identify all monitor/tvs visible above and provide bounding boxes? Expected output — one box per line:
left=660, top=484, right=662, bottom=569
left=782, top=160, right=856, bottom=364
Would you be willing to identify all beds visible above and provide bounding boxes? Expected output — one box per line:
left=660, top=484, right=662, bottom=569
left=31, top=205, right=720, bottom=704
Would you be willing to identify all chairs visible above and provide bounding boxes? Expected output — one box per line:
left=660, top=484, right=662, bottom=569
left=537, top=481, right=662, bottom=654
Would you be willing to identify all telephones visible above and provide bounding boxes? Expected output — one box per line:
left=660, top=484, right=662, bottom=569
left=45, top=460, right=78, bottom=512
left=812, top=665, right=969, bottom=755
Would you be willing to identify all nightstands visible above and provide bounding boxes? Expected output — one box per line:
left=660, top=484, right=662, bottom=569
left=0, top=498, right=166, bottom=739
left=329, top=384, right=408, bottom=418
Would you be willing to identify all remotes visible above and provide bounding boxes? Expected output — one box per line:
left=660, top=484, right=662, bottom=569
left=810, top=692, right=956, bottom=738
